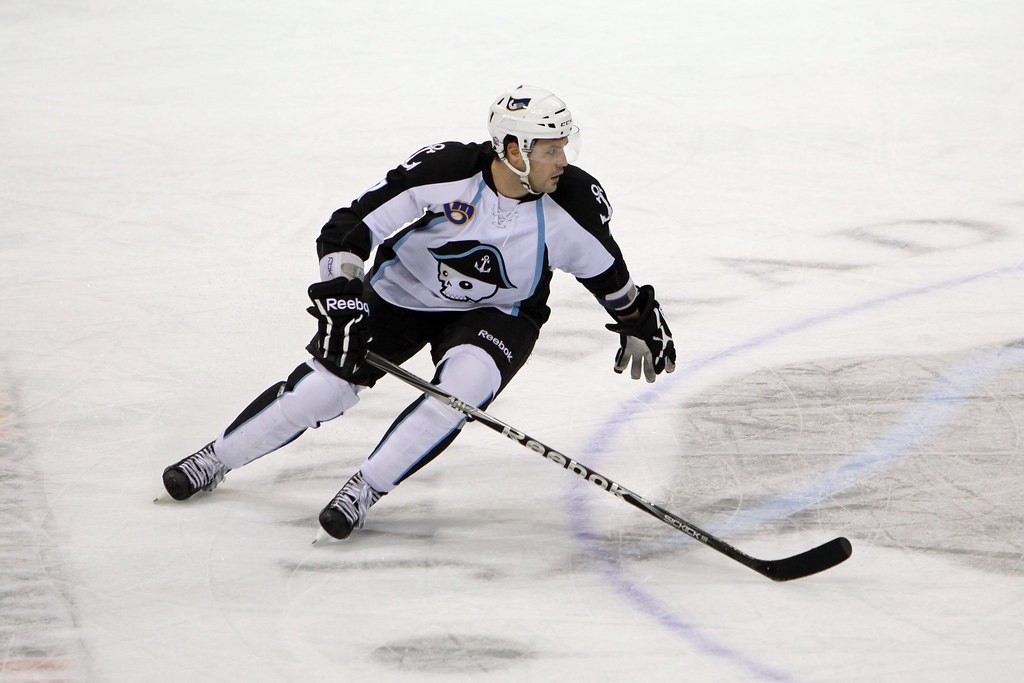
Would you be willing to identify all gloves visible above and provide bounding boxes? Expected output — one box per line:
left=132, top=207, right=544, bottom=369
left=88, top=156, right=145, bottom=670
left=604, top=285, right=676, bottom=382
left=306, top=276, right=373, bottom=373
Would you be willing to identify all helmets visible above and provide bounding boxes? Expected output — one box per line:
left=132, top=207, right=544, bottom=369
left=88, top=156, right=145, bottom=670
left=486, top=84, right=574, bottom=157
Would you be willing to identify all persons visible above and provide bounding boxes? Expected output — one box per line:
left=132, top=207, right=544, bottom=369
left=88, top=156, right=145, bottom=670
left=154, top=84, right=676, bottom=545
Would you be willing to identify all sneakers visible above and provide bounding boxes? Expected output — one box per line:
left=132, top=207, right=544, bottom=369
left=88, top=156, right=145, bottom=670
left=152, top=438, right=229, bottom=502
left=311, top=470, right=390, bottom=545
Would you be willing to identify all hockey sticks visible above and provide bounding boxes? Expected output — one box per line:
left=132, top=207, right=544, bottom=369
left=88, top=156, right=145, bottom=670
left=364, top=350, right=854, bottom=583
